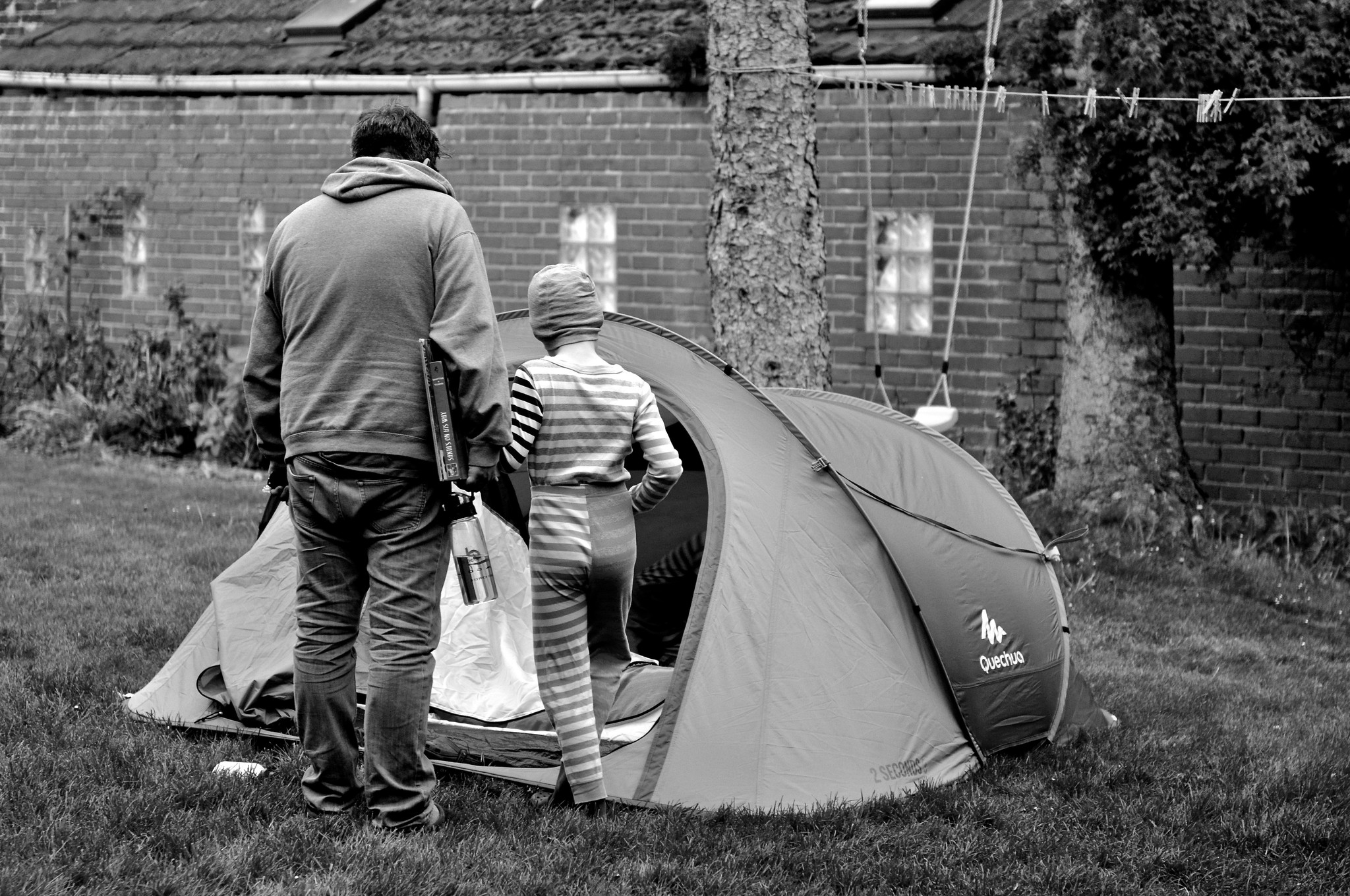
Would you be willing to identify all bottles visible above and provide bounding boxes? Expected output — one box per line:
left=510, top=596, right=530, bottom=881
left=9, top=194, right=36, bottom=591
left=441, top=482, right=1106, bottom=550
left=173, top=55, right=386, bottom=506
left=449, top=491, right=501, bottom=606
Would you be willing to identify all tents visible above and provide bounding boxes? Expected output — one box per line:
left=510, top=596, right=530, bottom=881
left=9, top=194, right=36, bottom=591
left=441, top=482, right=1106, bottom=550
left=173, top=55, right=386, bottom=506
left=121, top=307, right=1105, bottom=821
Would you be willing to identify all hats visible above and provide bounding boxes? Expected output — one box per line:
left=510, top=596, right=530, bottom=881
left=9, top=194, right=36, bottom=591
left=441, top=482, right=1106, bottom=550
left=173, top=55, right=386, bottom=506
left=528, top=262, right=604, bottom=353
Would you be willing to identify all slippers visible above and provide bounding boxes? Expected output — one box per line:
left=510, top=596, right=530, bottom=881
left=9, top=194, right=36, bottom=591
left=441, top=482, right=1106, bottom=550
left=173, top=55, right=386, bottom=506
left=529, top=790, right=553, bottom=814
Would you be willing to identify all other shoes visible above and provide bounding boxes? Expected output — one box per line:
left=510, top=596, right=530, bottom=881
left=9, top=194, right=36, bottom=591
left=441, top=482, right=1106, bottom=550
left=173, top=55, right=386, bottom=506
left=394, top=802, right=457, bottom=838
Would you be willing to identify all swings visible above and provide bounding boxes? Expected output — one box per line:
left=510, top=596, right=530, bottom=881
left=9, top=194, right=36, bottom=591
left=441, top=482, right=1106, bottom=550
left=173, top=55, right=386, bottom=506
left=855, top=0, right=1004, bottom=430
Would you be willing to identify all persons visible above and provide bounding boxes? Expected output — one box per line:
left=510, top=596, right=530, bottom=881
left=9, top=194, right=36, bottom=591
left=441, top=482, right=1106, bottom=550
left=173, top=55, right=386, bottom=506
left=242, top=106, right=512, bottom=833
left=490, top=260, right=682, bottom=821
